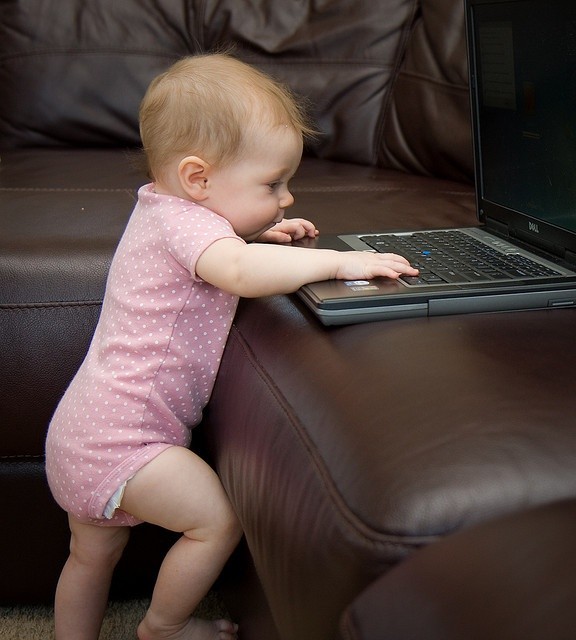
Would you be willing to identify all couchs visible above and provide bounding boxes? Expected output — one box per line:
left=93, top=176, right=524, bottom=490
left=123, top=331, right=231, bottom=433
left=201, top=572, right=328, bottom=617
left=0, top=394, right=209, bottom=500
left=1, top=0, right=575, bottom=638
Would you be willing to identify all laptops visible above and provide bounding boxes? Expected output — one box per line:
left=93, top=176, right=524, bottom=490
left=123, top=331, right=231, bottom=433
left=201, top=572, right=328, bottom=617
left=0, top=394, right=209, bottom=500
left=280, top=0, right=575, bottom=326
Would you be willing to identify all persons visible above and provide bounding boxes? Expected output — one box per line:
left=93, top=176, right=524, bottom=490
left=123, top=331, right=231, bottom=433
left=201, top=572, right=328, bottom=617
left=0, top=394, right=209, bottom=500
left=45, top=51, right=420, bottom=639
